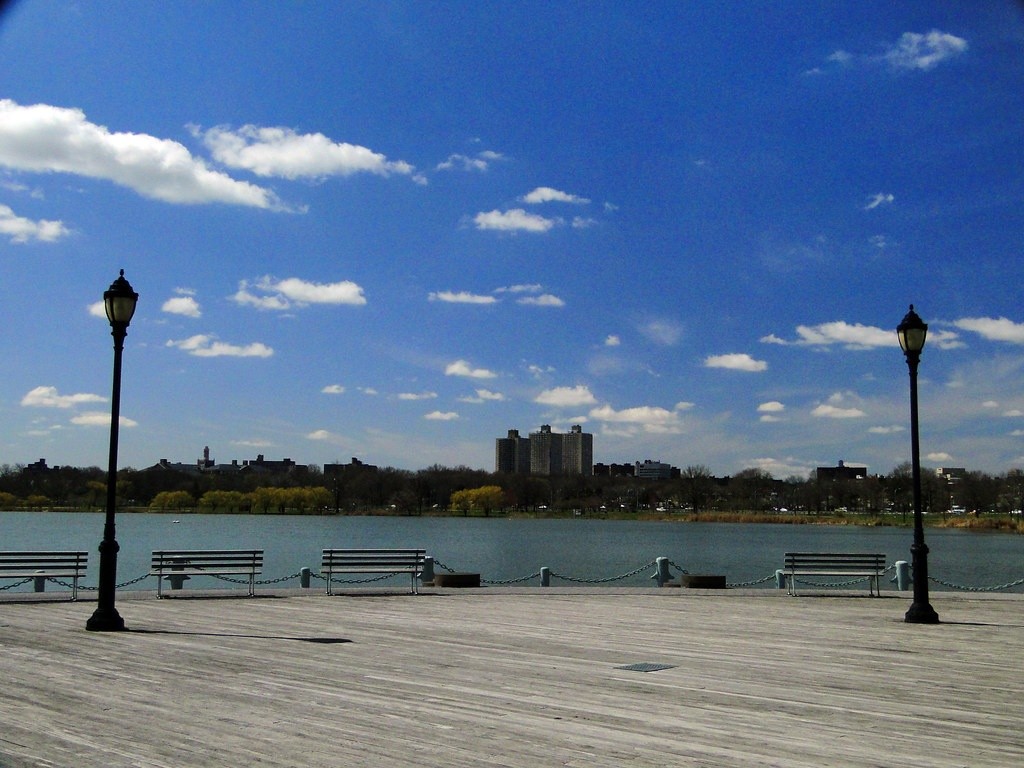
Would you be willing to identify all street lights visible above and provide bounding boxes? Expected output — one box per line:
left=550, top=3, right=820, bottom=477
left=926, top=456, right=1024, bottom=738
left=84, top=267, right=140, bottom=632
left=895, top=304, right=939, bottom=623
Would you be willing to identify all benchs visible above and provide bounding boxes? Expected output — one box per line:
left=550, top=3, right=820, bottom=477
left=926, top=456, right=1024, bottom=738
left=150, top=549, right=264, bottom=600
left=779, top=552, right=886, bottom=598
left=319, top=548, right=428, bottom=596
left=0, top=550, right=90, bottom=601
